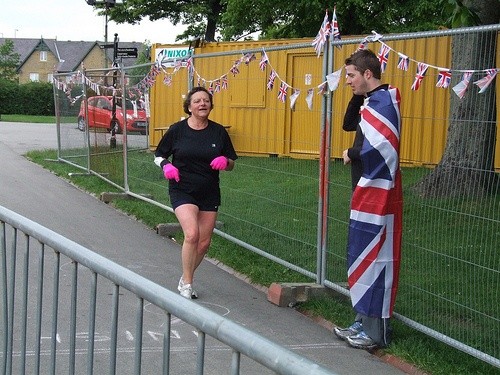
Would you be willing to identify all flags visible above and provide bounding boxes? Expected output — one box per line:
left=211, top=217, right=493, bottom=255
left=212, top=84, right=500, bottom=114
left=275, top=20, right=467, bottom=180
left=377, top=44, right=390, bottom=72
left=312, top=14, right=331, bottom=58
left=306, top=89, right=313, bottom=111
left=266, top=70, right=276, bottom=92
left=317, top=81, right=328, bottom=95
left=474, top=68, right=498, bottom=93
left=288, top=88, right=299, bottom=111
left=411, top=62, right=427, bottom=91
left=355, top=31, right=384, bottom=53
left=327, top=70, right=342, bottom=91
left=452, top=72, right=473, bottom=98
left=397, top=52, right=409, bottom=71
left=436, top=67, right=451, bottom=88
left=53, top=50, right=268, bottom=103
left=329, top=13, right=342, bottom=50
left=276, top=81, right=288, bottom=104
left=347, top=88, right=402, bottom=319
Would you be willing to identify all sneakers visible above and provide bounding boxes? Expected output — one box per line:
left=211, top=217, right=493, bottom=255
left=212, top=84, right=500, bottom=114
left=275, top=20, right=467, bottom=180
left=345, top=331, right=387, bottom=349
left=181, top=286, right=192, bottom=300
left=333, top=322, right=364, bottom=341
left=177, top=277, right=198, bottom=299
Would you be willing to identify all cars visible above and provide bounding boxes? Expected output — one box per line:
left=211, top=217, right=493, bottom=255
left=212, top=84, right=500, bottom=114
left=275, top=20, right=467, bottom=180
left=78, top=95, right=150, bottom=134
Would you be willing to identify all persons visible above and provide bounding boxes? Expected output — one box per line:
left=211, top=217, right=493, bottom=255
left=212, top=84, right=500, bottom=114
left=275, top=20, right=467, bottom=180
left=334, top=48, right=403, bottom=349
left=137, top=96, right=146, bottom=116
left=155, top=87, right=239, bottom=299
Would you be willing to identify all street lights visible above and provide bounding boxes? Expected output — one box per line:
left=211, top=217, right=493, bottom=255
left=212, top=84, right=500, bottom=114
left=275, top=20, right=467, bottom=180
left=15, top=28, right=19, bottom=37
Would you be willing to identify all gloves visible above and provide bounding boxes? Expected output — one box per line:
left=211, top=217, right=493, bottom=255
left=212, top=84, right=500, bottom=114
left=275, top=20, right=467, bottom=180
left=160, top=159, right=179, bottom=182
left=210, top=156, right=230, bottom=170
